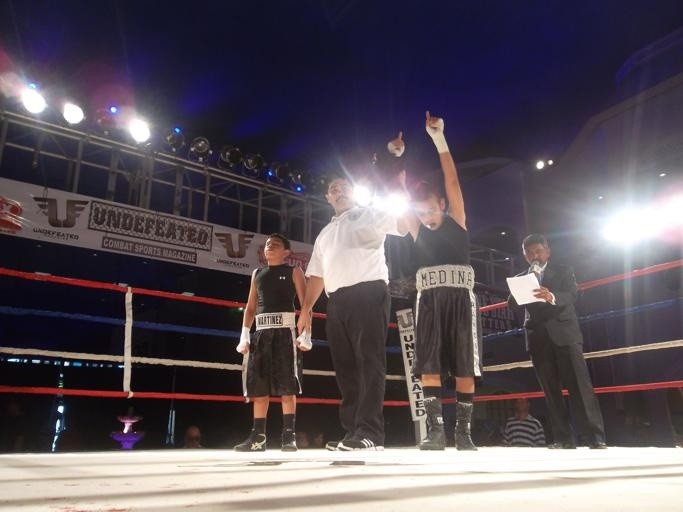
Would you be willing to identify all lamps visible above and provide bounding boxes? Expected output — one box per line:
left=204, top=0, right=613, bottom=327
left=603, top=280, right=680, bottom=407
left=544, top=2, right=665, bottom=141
left=158, top=117, right=333, bottom=195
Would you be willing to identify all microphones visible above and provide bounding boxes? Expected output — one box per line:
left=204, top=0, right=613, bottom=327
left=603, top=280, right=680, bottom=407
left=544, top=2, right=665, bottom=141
left=528, top=261, right=542, bottom=277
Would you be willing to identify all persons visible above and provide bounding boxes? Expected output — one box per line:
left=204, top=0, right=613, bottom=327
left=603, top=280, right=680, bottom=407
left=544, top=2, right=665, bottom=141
left=1, top=392, right=34, bottom=451
left=396, top=111, right=482, bottom=450
left=62, top=409, right=89, bottom=450
left=296, top=431, right=324, bottom=449
left=233, top=234, right=302, bottom=450
left=499, top=396, right=547, bottom=447
left=294, top=131, right=413, bottom=450
left=183, top=426, right=204, bottom=448
left=507, top=232, right=607, bottom=448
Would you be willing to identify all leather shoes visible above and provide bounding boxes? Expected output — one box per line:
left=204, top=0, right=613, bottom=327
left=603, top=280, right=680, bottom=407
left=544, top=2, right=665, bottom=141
left=546, top=443, right=606, bottom=448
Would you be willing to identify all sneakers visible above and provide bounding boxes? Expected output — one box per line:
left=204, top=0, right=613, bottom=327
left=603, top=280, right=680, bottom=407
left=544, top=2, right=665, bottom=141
left=234, top=432, right=266, bottom=451
left=326, top=436, right=384, bottom=451
left=281, top=431, right=298, bottom=451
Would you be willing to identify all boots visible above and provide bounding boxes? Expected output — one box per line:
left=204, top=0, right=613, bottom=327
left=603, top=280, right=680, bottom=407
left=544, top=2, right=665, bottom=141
left=419, top=400, right=445, bottom=449
left=454, top=403, right=479, bottom=450
